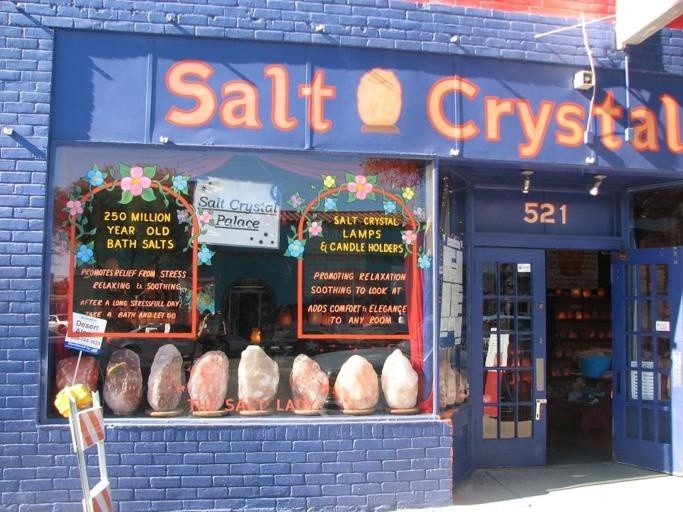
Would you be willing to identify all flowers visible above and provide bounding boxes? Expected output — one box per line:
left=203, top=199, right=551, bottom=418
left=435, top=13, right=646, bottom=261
left=63, top=163, right=218, bottom=267
left=283, top=169, right=431, bottom=272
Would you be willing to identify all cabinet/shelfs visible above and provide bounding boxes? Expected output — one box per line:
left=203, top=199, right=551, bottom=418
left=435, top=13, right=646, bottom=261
left=547, top=290, right=611, bottom=386
left=551, top=386, right=613, bottom=434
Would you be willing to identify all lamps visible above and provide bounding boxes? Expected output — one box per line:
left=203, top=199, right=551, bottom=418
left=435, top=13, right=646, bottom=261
left=588, top=181, right=601, bottom=196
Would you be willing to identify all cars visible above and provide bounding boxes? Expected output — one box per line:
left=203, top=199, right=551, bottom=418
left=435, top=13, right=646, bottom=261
left=105, top=325, right=198, bottom=360
left=49, top=312, right=68, bottom=335
